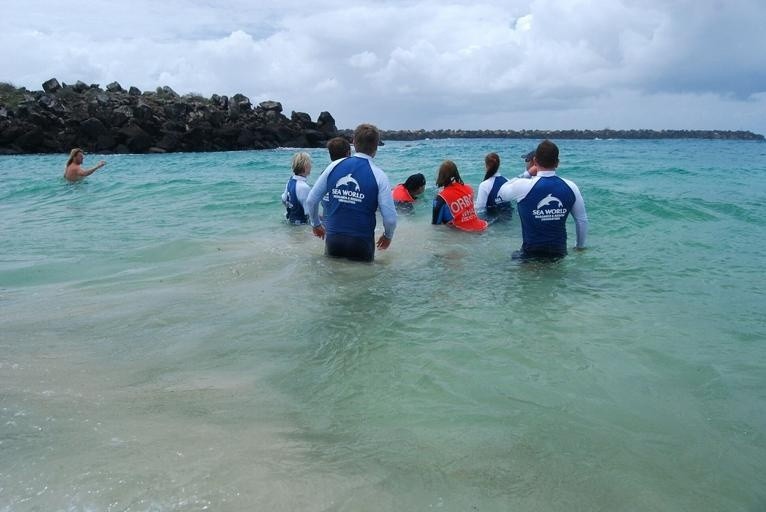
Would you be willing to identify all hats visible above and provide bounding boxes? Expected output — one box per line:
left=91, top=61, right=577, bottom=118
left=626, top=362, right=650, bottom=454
left=520, top=149, right=536, bottom=161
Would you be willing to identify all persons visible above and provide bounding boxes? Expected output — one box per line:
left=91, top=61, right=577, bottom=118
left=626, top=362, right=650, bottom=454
left=64, top=148, right=106, bottom=181
left=391, top=172, right=426, bottom=215
left=432, top=160, right=488, bottom=231
left=520, top=150, right=536, bottom=171
left=319, top=137, right=351, bottom=230
left=306, top=123, right=398, bottom=263
left=496, top=140, right=587, bottom=259
left=281, top=152, right=323, bottom=226
left=475, top=152, right=510, bottom=209
